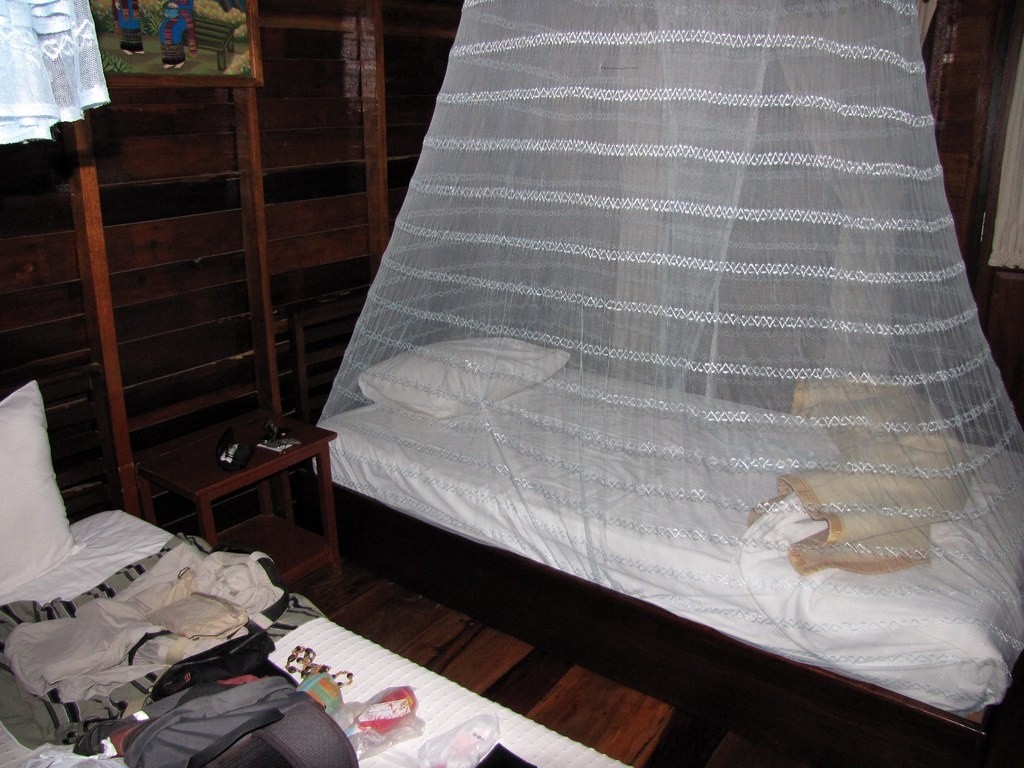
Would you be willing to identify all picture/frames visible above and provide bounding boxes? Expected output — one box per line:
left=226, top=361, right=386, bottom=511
left=88, top=0, right=265, bottom=89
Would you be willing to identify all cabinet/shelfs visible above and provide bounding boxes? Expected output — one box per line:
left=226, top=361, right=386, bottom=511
left=0, top=0, right=462, bottom=539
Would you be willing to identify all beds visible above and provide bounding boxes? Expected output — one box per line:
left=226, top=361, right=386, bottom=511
left=290, top=296, right=1024, bottom=768
left=0, top=364, right=633, bottom=768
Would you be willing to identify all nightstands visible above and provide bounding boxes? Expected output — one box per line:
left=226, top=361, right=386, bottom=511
left=136, top=412, right=339, bottom=592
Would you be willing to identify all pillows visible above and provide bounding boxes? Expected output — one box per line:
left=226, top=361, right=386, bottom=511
left=0, top=379, right=85, bottom=600
left=357, top=336, right=571, bottom=417
